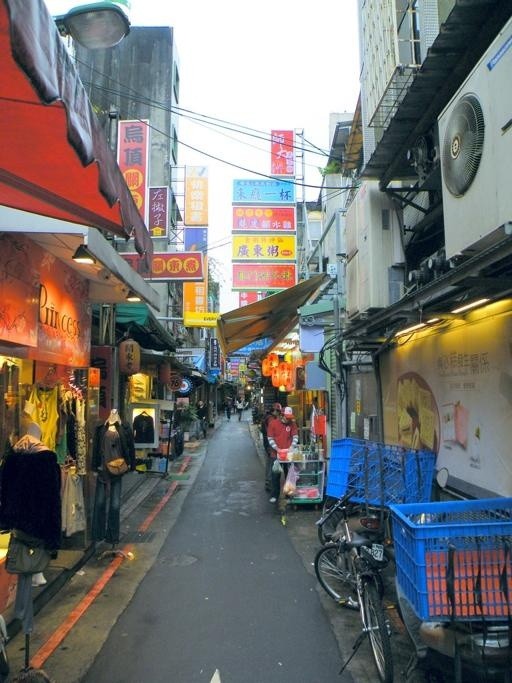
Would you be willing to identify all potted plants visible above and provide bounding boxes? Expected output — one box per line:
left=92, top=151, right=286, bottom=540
left=178, top=403, right=200, bottom=441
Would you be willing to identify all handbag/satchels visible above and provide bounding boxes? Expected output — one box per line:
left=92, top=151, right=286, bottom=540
left=4, top=535, right=53, bottom=574
left=135, top=454, right=169, bottom=472
left=309, top=406, right=327, bottom=442
left=106, top=457, right=128, bottom=476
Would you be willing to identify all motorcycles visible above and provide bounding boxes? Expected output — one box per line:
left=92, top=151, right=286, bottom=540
left=380, top=471, right=512, bottom=682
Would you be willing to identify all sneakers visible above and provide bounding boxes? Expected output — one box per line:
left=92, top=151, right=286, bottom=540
left=264, top=480, right=278, bottom=503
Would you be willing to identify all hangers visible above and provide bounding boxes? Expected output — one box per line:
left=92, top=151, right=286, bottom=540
left=140, top=410, right=150, bottom=416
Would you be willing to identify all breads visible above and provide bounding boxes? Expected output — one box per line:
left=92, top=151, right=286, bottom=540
left=396, top=378, right=435, bottom=450
left=284, top=482, right=295, bottom=495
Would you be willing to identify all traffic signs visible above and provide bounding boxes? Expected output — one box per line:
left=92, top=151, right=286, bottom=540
left=275, top=448, right=326, bottom=510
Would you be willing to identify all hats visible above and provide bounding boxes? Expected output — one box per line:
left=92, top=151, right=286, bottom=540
left=272, top=402, right=295, bottom=419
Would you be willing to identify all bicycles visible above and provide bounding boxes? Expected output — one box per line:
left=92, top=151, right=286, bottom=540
left=310, top=487, right=395, bottom=681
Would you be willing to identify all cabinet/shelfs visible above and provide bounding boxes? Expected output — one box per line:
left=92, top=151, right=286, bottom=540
left=277, top=458, right=326, bottom=510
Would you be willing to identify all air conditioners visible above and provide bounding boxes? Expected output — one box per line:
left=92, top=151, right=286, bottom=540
left=435, top=15, right=512, bottom=262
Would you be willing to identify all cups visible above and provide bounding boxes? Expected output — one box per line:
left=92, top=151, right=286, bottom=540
left=438, top=401, right=482, bottom=471
left=278, top=449, right=288, bottom=461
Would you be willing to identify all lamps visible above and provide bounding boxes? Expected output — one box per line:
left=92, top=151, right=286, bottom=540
left=71, top=243, right=97, bottom=265
left=53, top=1, right=132, bottom=49
left=125, top=289, right=142, bottom=302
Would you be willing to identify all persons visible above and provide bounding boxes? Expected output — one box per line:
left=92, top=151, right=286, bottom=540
left=259, top=401, right=283, bottom=490
left=90, top=408, right=140, bottom=545
left=224, top=396, right=233, bottom=422
left=1, top=420, right=63, bottom=636
left=266, top=405, right=300, bottom=505
left=196, top=399, right=207, bottom=439
left=235, top=397, right=245, bottom=421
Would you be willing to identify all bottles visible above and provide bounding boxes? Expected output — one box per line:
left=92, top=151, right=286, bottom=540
left=288, top=444, right=324, bottom=461
left=159, top=417, right=177, bottom=438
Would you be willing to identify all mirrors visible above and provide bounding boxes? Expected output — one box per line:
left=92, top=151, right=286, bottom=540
left=130, top=402, right=160, bottom=448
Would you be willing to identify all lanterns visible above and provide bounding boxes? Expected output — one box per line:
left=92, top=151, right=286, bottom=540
left=119, top=337, right=141, bottom=375
left=272, top=353, right=314, bottom=391
left=159, top=361, right=171, bottom=385
left=267, top=352, right=279, bottom=368
left=261, top=358, right=272, bottom=377
